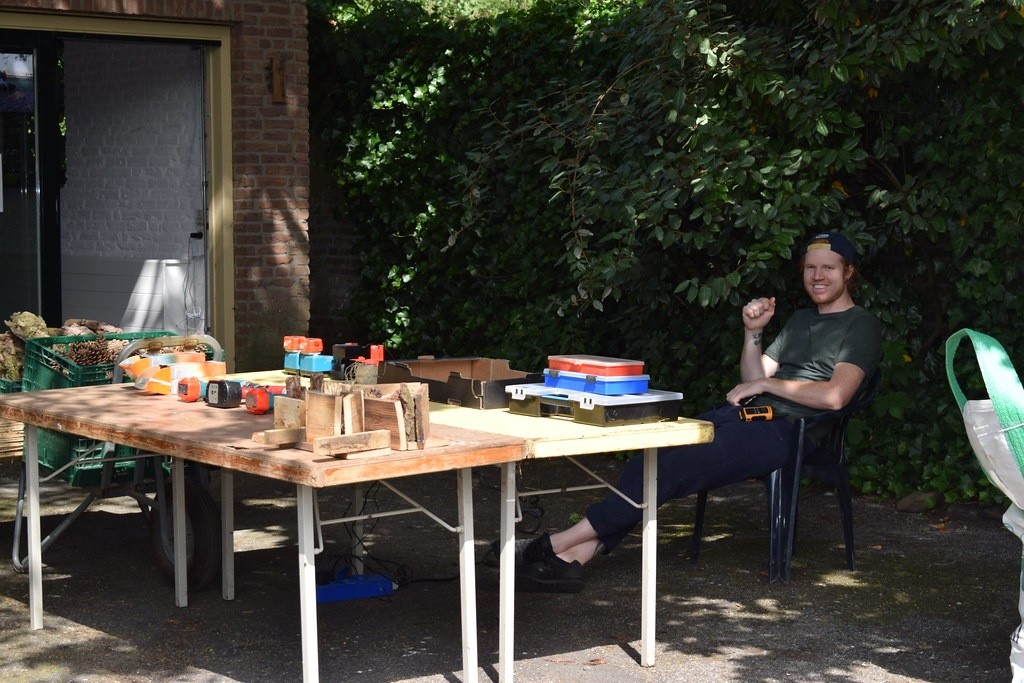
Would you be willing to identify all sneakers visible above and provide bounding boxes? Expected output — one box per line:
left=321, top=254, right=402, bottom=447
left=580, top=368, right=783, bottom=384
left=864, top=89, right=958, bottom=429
left=515, top=560, right=585, bottom=594
left=491, top=532, right=556, bottom=567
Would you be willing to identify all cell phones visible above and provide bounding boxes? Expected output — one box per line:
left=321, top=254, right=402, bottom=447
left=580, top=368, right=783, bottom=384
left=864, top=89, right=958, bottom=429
left=738, top=394, right=758, bottom=406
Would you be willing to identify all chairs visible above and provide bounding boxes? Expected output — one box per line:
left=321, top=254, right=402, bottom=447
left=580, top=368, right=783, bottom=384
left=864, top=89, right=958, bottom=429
left=688, top=364, right=882, bottom=584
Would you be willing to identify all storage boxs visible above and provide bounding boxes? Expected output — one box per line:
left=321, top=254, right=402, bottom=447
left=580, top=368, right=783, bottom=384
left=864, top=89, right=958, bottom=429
left=542, top=368, right=652, bottom=395
left=20, top=332, right=224, bottom=484
left=375, top=356, right=546, bottom=410
left=546, top=353, right=645, bottom=377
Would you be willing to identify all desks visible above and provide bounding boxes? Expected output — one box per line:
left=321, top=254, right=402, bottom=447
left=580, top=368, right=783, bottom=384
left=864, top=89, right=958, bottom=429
left=0, top=382, right=527, bottom=683
left=213, top=365, right=714, bottom=683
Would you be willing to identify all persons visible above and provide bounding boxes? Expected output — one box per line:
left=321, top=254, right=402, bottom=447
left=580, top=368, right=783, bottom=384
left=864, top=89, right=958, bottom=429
left=490, top=232, right=887, bottom=592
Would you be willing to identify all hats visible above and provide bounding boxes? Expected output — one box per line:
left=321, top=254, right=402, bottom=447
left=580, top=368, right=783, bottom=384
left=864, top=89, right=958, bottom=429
left=801, top=231, right=856, bottom=267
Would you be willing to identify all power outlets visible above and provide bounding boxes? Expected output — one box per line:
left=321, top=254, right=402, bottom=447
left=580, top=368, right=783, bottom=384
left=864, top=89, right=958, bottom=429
left=191, top=231, right=205, bottom=257
